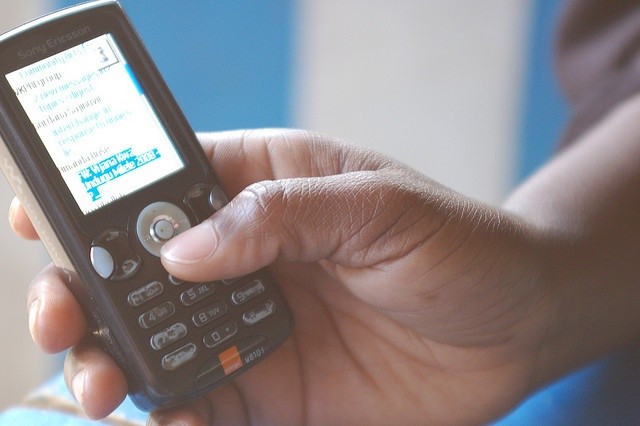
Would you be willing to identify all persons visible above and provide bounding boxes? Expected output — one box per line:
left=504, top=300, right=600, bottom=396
left=5, top=2, right=638, bottom=425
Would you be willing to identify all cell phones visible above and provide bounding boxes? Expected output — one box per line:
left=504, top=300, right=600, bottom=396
left=2, top=0, right=294, bottom=413
